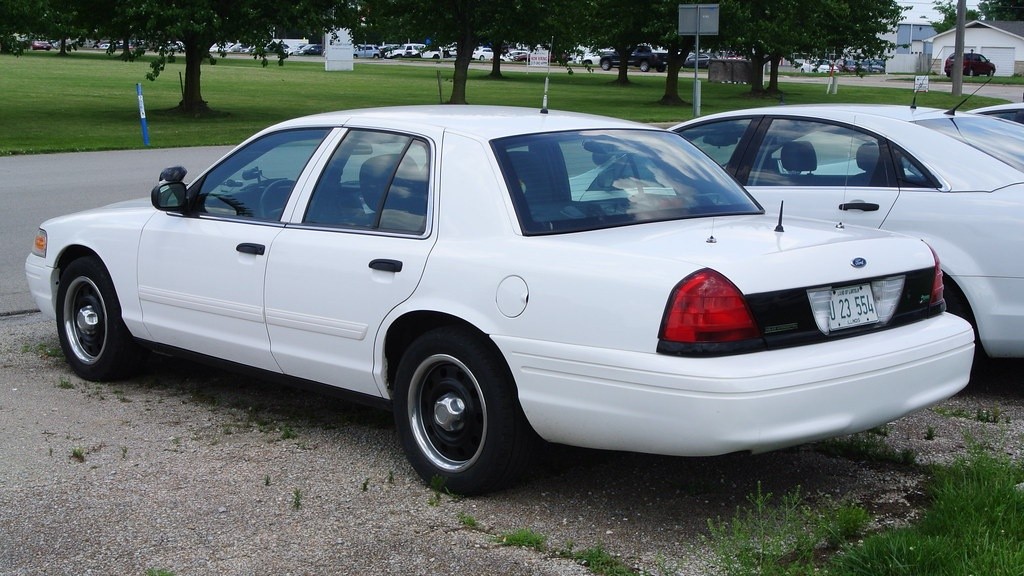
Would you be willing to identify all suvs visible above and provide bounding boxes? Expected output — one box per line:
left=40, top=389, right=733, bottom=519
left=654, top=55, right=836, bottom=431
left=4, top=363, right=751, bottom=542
left=600, top=44, right=669, bottom=72
left=945, top=53, right=997, bottom=78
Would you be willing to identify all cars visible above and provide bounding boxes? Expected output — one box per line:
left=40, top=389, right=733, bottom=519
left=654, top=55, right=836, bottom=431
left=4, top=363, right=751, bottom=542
left=568, top=103, right=1024, bottom=385
left=352, top=45, right=382, bottom=60
left=783, top=102, right=1024, bottom=178
left=22, top=103, right=977, bottom=492
left=514, top=51, right=569, bottom=63
left=834, top=60, right=885, bottom=74
left=472, top=48, right=503, bottom=61
left=379, top=43, right=458, bottom=59
left=284, top=43, right=325, bottom=56
left=575, top=47, right=615, bottom=65
left=683, top=52, right=747, bottom=70
left=32, top=40, right=52, bottom=51
left=500, top=51, right=527, bottom=62
left=801, top=59, right=839, bottom=74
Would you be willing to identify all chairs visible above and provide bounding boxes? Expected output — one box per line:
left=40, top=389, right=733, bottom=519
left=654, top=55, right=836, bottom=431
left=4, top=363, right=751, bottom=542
left=777, top=141, right=836, bottom=184
left=505, top=150, right=587, bottom=222
left=843, top=143, right=880, bottom=186
left=348, top=152, right=422, bottom=233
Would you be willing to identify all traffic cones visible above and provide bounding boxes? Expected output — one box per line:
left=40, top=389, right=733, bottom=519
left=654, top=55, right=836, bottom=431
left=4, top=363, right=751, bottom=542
left=99, top=41, right=288, bottom=53
